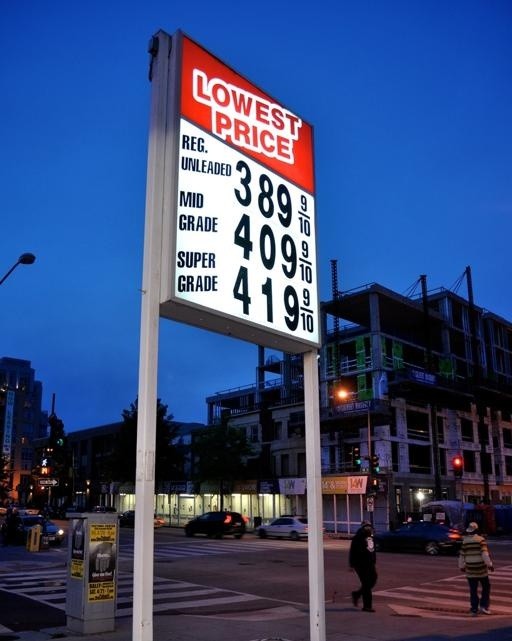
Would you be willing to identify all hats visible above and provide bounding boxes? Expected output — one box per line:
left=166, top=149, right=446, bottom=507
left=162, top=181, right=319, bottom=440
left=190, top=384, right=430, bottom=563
left=360, top=520, right=371, bottom=528
left=466, top=522, right=478, bottom=532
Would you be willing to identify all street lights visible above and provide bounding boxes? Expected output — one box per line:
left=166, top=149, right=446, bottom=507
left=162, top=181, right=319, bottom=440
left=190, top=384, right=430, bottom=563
left=0, top=251, right=36, bottom=284
left=336, top=386, right=377, bottom=535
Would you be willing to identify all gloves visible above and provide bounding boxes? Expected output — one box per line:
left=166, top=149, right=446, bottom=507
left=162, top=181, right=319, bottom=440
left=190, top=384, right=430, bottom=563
left=488, top=567, right=494, bottom=572
left=461, top=568, right=465, bottom=572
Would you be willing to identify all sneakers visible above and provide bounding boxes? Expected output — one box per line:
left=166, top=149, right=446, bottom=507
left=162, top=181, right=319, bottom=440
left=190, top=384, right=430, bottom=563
left=352, top=591, right=376, bottom=613
left=469, top=607, right=491, bottom=616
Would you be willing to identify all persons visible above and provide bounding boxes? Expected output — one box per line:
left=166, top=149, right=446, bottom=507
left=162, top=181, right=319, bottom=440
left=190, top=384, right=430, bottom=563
left=458, top=522, right=494, bottom=616
left=348, top=520, right=377, bottom=612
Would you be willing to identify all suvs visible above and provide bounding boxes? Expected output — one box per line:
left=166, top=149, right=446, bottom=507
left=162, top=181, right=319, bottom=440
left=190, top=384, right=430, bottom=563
left=14, top=514, right=65, bottom=547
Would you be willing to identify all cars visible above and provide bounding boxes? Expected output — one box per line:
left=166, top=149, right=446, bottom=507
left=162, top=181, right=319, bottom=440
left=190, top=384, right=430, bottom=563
left=182, top=510, right=249, bottom=539
left=0, top=502, right=167, bottom=529
left=255, top=516, right=326, bottom=539
left=372, top=521, right=466, bottom=555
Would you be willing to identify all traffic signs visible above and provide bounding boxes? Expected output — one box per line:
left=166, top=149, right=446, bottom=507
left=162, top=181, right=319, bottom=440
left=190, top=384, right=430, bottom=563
left=37, top=477, right=59, bottom=486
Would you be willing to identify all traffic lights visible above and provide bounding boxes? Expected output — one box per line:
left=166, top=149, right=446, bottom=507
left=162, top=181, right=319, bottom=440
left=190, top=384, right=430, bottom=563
left=41, top=457, right=48, bottom=473
left=371, top=455, right=380, bottom=474
left=353, top=446, right=362, bottom=465
left=452, top=455, right=463, bottom=478
left=51, top=418, right=64, bottom=446
left=371, top=479, right=378, bottom=487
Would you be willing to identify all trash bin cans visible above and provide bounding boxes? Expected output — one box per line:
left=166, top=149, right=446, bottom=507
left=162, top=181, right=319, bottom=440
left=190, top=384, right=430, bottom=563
left=254, top=516, right=262, bottom=528
